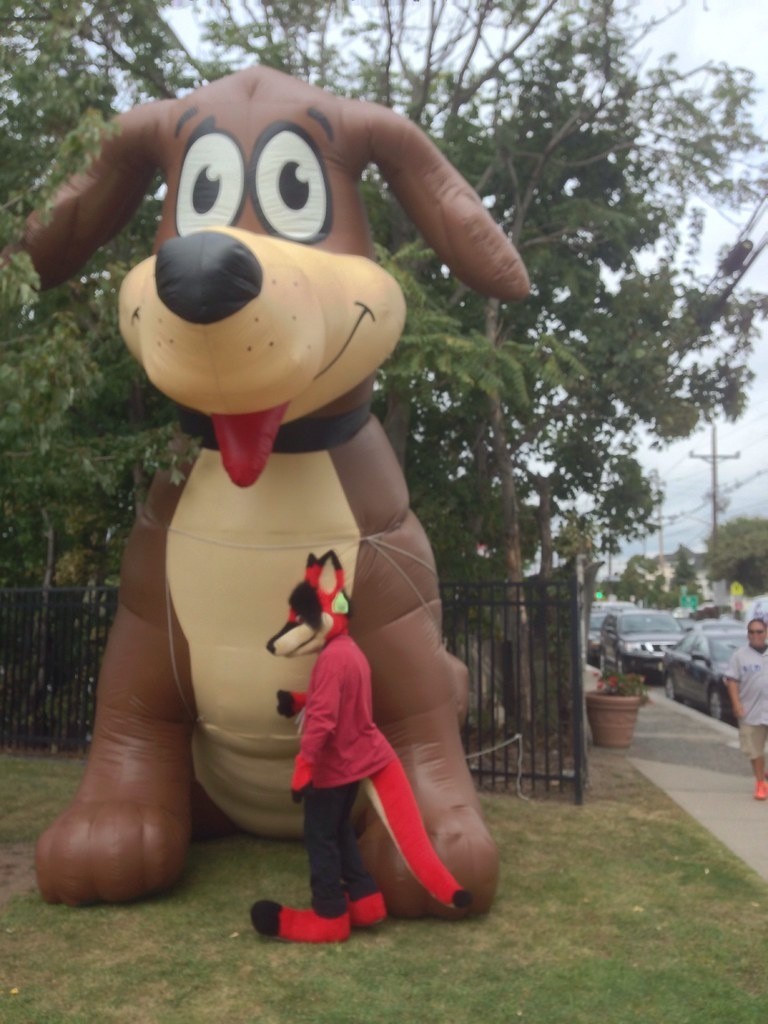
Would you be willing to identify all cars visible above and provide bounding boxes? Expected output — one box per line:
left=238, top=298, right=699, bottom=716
left=661, top=621, right=754, bottom=725
left=587, top=600, right=639, bottom=665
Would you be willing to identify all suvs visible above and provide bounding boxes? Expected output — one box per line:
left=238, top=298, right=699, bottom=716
left=597, top=608, right=685, bottom=686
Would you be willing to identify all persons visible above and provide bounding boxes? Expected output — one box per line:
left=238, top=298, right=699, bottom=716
left=722, top=618, right=768, bottom=802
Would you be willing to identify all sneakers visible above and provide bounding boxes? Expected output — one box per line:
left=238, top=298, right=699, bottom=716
left=754, top=781, right=768, bottom=800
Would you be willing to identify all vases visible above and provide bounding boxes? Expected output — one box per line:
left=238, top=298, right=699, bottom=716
left=587, top=689, right=642, bottom=750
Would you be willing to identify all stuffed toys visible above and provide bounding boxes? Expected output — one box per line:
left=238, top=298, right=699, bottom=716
left=245, top=549, right=477, bottom=940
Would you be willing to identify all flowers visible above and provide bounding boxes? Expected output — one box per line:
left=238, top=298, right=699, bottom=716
left=592, top=665, right=655, bottom=707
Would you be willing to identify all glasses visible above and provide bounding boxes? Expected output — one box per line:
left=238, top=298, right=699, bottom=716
left=747, top=629, right=766, bottom=635
